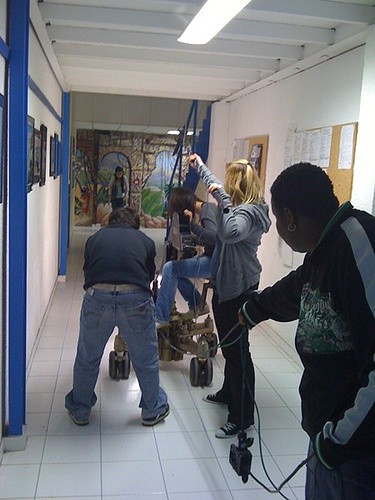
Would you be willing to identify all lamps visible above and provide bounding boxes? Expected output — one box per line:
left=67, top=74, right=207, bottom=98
left=177, top=0, right=252, bottom=45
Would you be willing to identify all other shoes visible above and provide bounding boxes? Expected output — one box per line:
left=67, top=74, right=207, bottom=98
left=188, top=302, right=210, bottom=316
left=154, top=319, right=170, bottom=329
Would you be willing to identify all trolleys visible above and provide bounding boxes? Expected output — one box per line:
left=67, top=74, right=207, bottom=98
left=108, top=253, right=218, bottom=385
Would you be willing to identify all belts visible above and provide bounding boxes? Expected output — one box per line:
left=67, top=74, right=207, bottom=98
left=86, top=282, right=142, bottom=297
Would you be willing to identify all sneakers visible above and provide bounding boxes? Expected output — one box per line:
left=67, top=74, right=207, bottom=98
left=67, top=408, right=89, bottom=426
left=143, top=403, right=170, bottom=426
left=202, top=393, right=228, bottom=404
left=215, top=422, right=251, bottom=438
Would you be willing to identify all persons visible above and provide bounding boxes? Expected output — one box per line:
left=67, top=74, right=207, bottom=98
left=190, top=154, right=272, bottom=437
left=65, top=208, right=170, bottom=425
left=155, top=187, right=219, bottom=319
left=238, top=162, right=375, bottom=500
left=109, top=166, right=129, bottom=210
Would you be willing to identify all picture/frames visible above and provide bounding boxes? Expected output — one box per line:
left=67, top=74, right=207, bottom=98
left=28, top=115, right=63, bottom=195
